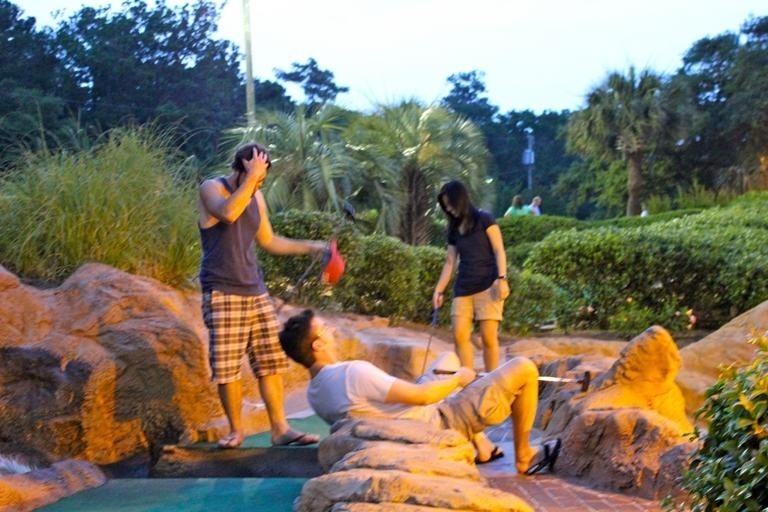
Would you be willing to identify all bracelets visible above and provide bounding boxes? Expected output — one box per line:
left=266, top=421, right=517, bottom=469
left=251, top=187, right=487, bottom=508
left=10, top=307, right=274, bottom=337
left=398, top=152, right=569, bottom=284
left=497, top=275, right=507, bottom=280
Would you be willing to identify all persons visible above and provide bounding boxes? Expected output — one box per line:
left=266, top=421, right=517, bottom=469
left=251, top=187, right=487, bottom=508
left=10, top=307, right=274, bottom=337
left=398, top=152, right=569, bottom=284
left=639, top=202, right=649, bottom=217
left=528, top=196, right=542, bottom=216
left=198, top=145, right=345, bottom=447
left=278, top=310, right=562, bottom=477
left=419, top=180, right=510, bottom=372
left=504, top=195, right=534, bottom=217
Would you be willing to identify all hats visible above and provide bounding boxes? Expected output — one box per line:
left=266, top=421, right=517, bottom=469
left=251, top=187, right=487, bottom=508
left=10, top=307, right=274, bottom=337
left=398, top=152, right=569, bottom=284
left=315, top=239, right=345, bottom=284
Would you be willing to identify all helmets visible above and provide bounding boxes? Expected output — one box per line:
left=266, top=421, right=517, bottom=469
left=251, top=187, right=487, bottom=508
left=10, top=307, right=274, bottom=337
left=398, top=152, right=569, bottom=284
left=232, top=145, right=273, bottom=171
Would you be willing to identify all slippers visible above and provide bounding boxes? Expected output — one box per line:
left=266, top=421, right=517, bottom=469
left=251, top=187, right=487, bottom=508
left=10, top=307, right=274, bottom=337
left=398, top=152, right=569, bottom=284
left=272, top=433, right=318, bottom=447
left=526, top=439, right=559, bottom=475
left=474, top=445, right=505, bottom=465
left=216, top=435, right=242, bottom=450
